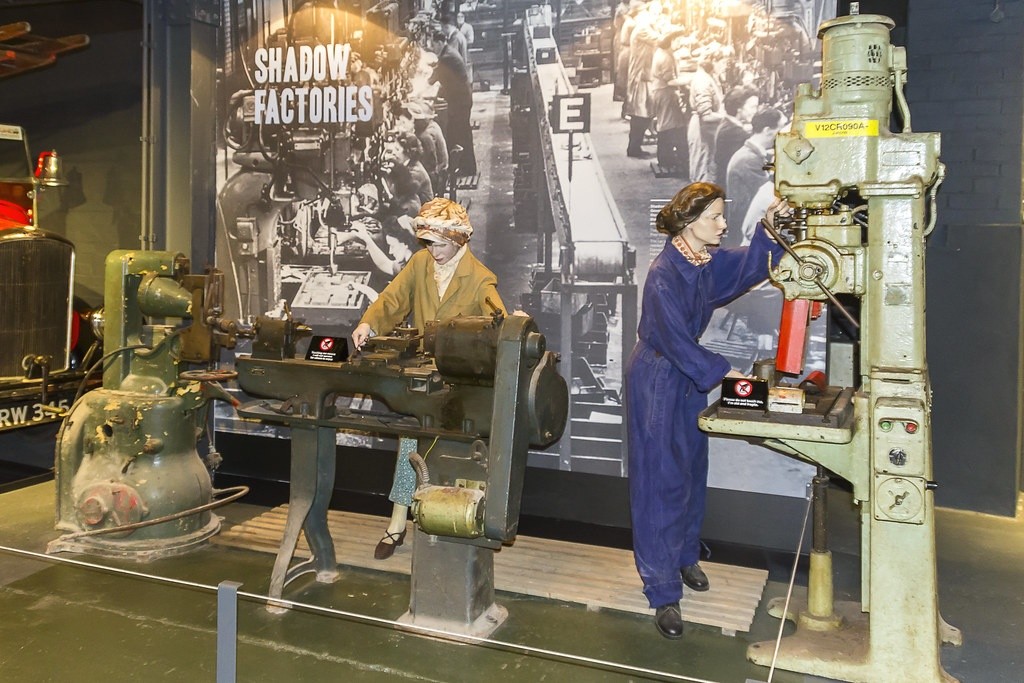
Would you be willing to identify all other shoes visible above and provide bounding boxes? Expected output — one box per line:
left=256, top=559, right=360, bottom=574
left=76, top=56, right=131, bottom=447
left=613, top=95, right=624, bottom=100
left=628, top=149, right=649, bottom=158
